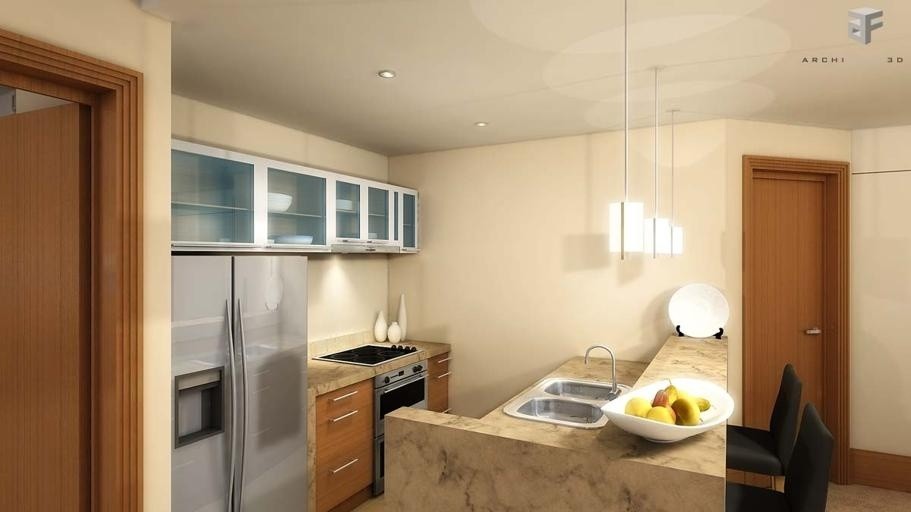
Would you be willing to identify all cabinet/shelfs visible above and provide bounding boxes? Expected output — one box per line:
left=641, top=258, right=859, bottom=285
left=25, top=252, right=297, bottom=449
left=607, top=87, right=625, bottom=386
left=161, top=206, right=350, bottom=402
left=172, top=139, right=263, bottom=248
left=427, top=349, right=450, bottom=413
left=317, top=373, right=374, bottom=512
left=389, top=183, right=418, bottom=252
left=261, top=157, right=331, bottom=254
left=328, top=170, right=394, bottom=244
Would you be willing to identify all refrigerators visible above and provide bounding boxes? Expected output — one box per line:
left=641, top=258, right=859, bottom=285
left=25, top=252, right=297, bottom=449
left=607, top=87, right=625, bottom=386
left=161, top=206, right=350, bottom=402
left=171, top=255, right=310, bottom=512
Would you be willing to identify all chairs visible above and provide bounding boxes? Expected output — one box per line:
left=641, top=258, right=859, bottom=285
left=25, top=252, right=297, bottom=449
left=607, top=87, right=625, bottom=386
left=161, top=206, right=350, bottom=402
left=726, top=363, right=803, bottom=491
left=727, top=402, right=835, bottom=511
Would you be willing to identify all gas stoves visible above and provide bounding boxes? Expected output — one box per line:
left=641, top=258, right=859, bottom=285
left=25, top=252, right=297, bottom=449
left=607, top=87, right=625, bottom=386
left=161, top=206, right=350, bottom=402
left=314, top=343, right=428, bottom=387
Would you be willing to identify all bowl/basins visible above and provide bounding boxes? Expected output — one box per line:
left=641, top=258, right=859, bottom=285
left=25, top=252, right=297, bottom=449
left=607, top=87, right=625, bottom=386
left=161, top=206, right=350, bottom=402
left=267, top=190, right=292, bottom=213
left=600, top=377, right=735, bottom=445
left=369, top=233, right=378, bottom=240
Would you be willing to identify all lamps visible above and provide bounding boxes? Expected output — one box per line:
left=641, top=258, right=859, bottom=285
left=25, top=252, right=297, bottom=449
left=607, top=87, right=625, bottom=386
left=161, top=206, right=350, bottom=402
left=663, top=108, right=684, bottom=257
left=608, top=1, right=646, bottom=259
left=645, top=66, right=667, bottom=257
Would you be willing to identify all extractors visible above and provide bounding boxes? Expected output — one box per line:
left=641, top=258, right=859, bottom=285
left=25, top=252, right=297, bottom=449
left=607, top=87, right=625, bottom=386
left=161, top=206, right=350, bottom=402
left=331, top=244, right=401, bottom=254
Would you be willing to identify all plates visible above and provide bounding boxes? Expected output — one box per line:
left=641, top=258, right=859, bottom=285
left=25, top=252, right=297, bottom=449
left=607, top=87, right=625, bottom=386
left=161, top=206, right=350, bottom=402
left=268, top=233, right=312, bottom=245
left=668, top=282, right=729, bottom=338
left=335, top=198, right=354, bottom=212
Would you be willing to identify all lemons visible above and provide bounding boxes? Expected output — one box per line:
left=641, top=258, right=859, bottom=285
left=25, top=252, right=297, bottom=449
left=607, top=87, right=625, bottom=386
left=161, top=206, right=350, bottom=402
left=624, top=383, right=711, bottom=426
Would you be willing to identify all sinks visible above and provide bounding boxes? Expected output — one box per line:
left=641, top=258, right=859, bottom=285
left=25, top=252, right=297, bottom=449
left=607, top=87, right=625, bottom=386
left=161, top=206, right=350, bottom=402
left=531, top=374, right=632, bottom=404
left=502, top=393, right=609, bottom=429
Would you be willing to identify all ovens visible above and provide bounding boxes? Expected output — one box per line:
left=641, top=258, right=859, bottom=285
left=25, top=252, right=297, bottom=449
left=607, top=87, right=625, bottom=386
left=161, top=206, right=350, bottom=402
left=373, top=373, right=429, bottom=499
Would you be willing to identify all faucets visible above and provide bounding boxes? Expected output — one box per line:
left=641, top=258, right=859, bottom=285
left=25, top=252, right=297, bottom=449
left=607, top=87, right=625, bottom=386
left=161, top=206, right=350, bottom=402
left=585, top=345, right=622, bottom=400
